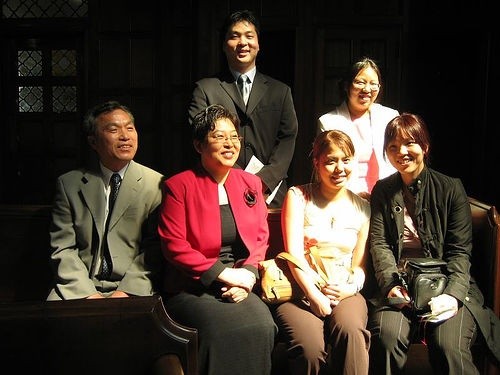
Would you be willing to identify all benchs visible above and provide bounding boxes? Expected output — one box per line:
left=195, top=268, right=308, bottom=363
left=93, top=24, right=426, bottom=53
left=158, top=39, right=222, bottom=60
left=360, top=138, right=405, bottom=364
left=0, top=195, right=500, bottom=375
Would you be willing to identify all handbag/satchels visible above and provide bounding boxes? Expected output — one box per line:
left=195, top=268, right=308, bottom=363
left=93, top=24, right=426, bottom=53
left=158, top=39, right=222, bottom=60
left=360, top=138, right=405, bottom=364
left=403, top=258, right=449, bottom=319
left=257, top=246, right=327, bottom=304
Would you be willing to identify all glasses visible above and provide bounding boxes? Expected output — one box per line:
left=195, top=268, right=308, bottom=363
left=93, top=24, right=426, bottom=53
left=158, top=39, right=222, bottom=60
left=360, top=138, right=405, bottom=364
left=206, top=133, right=243, bottom=143
left=350, top=80, right=381, bottom=91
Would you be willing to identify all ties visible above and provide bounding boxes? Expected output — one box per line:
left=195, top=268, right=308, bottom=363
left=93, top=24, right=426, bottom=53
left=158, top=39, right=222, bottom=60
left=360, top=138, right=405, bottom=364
left=101, top=173, right=122, bottom=281
left=240, top=74, right=250, bottom=105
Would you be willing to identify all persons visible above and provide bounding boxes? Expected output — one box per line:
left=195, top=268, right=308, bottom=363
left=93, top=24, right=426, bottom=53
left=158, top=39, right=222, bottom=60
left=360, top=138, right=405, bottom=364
left=183, top=10, right=297, bottom=208
left=155, top=104, right=280, bottom=375
left=45, top=100, right=166, bottom=301
left=266, top=131, right=372, bottom=375
left=319, top=57, right=401, bottom=193
left=366, top=113, right=500, bottom=375
